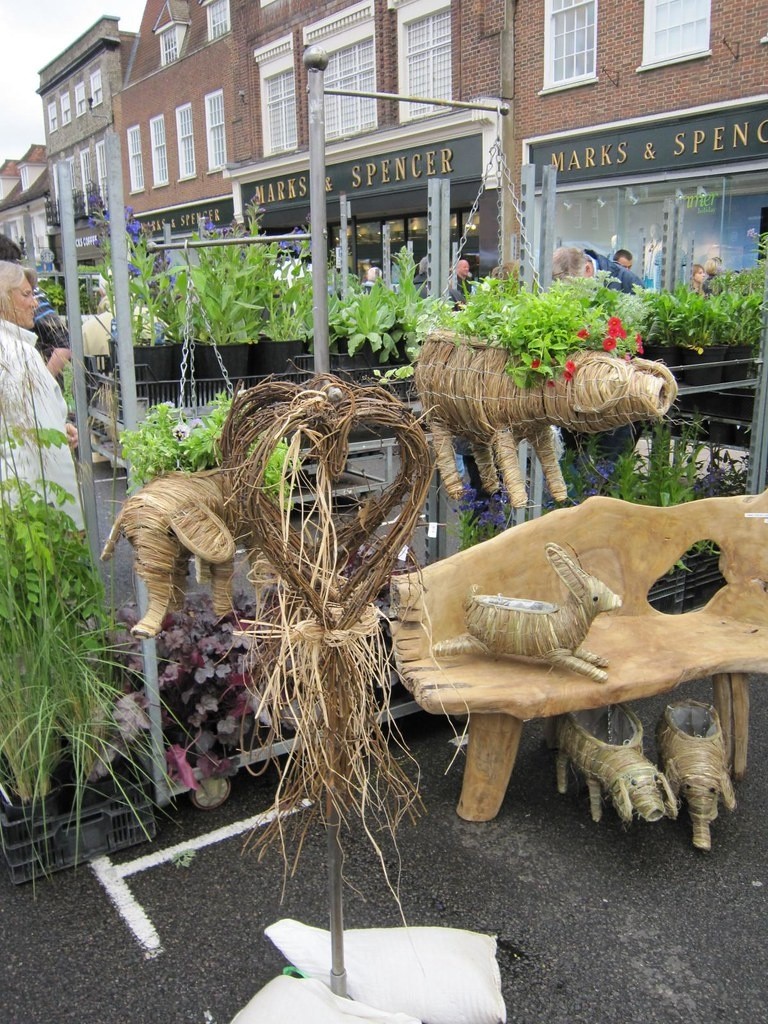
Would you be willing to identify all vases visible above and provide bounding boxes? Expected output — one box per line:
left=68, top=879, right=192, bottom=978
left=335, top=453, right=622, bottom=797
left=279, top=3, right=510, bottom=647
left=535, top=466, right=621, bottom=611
left=110, top=338, right=308, bottom=400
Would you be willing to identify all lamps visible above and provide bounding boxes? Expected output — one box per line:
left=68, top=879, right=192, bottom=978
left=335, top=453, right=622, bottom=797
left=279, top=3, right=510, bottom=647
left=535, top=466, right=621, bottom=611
left=629, top=194, right=640, bottom=206
left=87, top=97, right=108, bottom=118
left=697, top=184, right=708, bottom=197
left=674, top=186, right=683, bottom=200
left=595, top=198, right=607, bottom=208
left=237, top=90, right=246, bottom=105
left=563, top=202, right=574, bottom=211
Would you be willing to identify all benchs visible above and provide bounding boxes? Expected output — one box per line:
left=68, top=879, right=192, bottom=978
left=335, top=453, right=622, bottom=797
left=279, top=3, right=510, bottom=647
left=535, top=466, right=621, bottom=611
left=388, top=488, right=768, bottom=823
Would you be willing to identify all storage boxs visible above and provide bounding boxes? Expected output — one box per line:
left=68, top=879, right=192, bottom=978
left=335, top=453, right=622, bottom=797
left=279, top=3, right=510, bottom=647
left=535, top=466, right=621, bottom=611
left=1, top=749, right=157, bottom=887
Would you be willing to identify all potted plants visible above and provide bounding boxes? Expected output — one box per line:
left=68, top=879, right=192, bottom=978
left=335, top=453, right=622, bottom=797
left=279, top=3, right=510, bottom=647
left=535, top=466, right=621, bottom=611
left=329, top=254, right=443, bottom=377
left=630, top=276, right=767, bottom=386
left=1, top=283, right=128, bottom=820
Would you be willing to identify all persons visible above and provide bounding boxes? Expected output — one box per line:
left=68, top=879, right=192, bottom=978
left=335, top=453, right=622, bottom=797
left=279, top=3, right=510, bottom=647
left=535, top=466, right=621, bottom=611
left=362, top=267, right=382, bottom=294
left=691, top=257, right=740, bottom=300
left=552, top=247, right=646, bottom=296
left=449, top=259, right=500, bottom=312
left=83, top=268, right=172, bottom=355
left=413, top=256, right=428, bottom=299
left=0, top=235, right=85, bottom=539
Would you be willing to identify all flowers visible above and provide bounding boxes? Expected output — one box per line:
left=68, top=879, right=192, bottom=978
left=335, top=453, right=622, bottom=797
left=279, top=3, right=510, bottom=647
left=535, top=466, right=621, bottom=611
left=84, top=181, right=347, bottom=344
left=455, top=444, right=627, bottom=555
left=453, top=274, right=645, bottom=389
